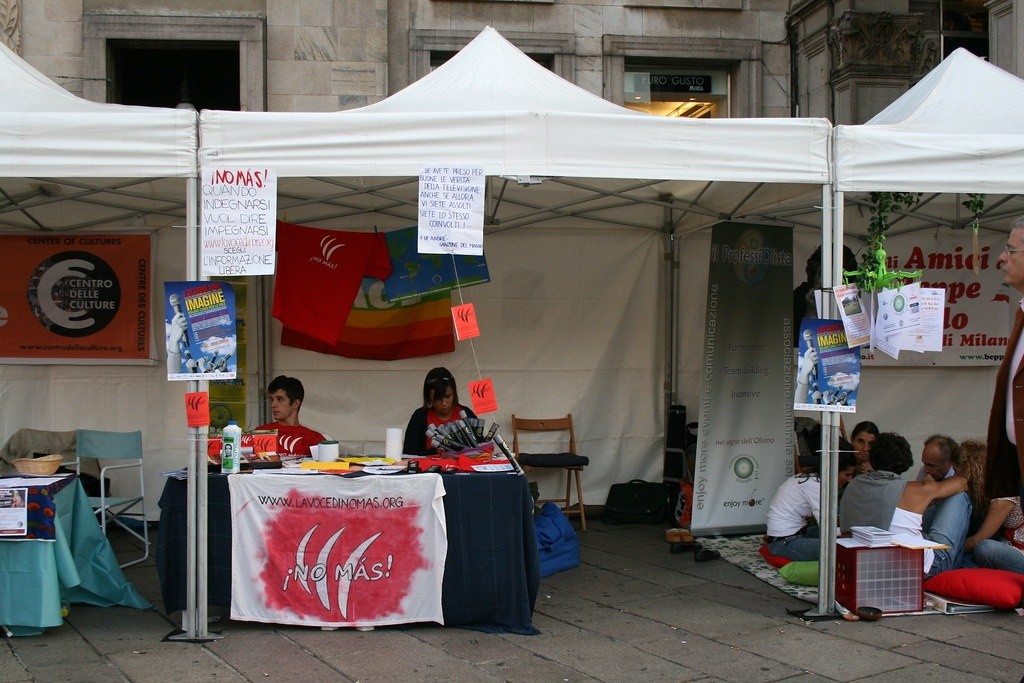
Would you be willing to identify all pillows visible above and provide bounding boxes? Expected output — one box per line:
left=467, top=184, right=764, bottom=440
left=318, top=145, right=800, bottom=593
left=922, top=567, right=1024, bottom=608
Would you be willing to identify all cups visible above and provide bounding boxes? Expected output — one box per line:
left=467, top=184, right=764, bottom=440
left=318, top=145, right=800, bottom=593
left=318, top=440, right=339, bottom=463
left=385, top=428, right=402, bottom=461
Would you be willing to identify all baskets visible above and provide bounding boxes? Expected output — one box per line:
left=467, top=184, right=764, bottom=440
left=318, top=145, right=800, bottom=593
left=13, top=454, right=63, bottom=475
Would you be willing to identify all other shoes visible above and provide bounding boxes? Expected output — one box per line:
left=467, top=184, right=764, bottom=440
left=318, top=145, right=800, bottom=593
left=680, top=528, right=694, bottom=542
left=694, top=550, right=720, bottom=563
left=670, top=541, right=701, bottom=554
left=666, top=528, right=682, bottom=543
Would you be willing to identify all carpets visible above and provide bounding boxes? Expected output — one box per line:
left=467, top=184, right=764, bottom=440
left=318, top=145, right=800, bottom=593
left=695, top=533, right=943, bottom=620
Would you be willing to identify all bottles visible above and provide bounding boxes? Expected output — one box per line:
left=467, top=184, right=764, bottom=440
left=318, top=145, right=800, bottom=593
left=221, top=421, right=242, bottom=474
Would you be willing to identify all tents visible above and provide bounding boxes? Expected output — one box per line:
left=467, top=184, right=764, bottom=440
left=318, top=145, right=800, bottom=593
left=0, top=26, right=1024, bottom=635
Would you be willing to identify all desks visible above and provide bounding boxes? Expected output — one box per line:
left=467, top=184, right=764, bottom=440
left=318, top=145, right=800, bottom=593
left=158, top=464, right=543, bottom=636
left=0, top=471, right=155, bottom=635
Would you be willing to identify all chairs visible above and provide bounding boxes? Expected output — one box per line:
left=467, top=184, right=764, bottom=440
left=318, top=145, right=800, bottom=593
left=66, top=428, right=151, bottom=571
left=513, top=414, right=590, bottom=534
left=0, top=428, right=113, bottom=514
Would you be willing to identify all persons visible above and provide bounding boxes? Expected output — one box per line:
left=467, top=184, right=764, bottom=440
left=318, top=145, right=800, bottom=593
left=839, top=433, right=972, bottom=579
left=851, top=421, right=879, bottom=474
left=254, top=375, right=333, bottom=458
left=402, top=366, right=478, bottom=456
left=916, top=434, right=958, bottom=482
left=982, top=217, right=1024, bottom=512
left=953, top=438, right=1024, bottom=575
left=764, top=441, right=858, bottom=562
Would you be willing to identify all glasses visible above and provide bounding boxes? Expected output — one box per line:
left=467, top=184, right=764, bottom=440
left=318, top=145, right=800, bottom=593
left=426, top=373, right=452, bottom=385
left=1004, top=246, right=1024, bottom=256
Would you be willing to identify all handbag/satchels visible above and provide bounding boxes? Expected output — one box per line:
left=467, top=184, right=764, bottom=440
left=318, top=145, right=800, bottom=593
left=533, top=502, right=580, bottom=577
left=600, top=479, right=678, bottom=525
